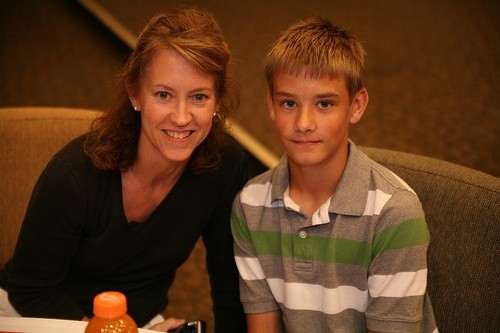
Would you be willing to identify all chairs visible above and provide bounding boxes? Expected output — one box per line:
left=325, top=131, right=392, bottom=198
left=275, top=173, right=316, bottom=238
left=354, top=147, right=500, bottom=333
left=1, top=108, right=216, bottom=333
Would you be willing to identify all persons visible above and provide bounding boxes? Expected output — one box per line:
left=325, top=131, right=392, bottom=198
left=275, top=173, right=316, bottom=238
left=0, top=7, right=250, bottom=333
left=230, top=18, right=439, bottom=333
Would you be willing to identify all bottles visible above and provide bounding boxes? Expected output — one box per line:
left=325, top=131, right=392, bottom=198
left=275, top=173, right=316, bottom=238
left=84, top=291, right=138, bottom=333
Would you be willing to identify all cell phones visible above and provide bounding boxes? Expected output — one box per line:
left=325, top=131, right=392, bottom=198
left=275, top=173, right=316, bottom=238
left=167, top=320, right=206, bottom=333
